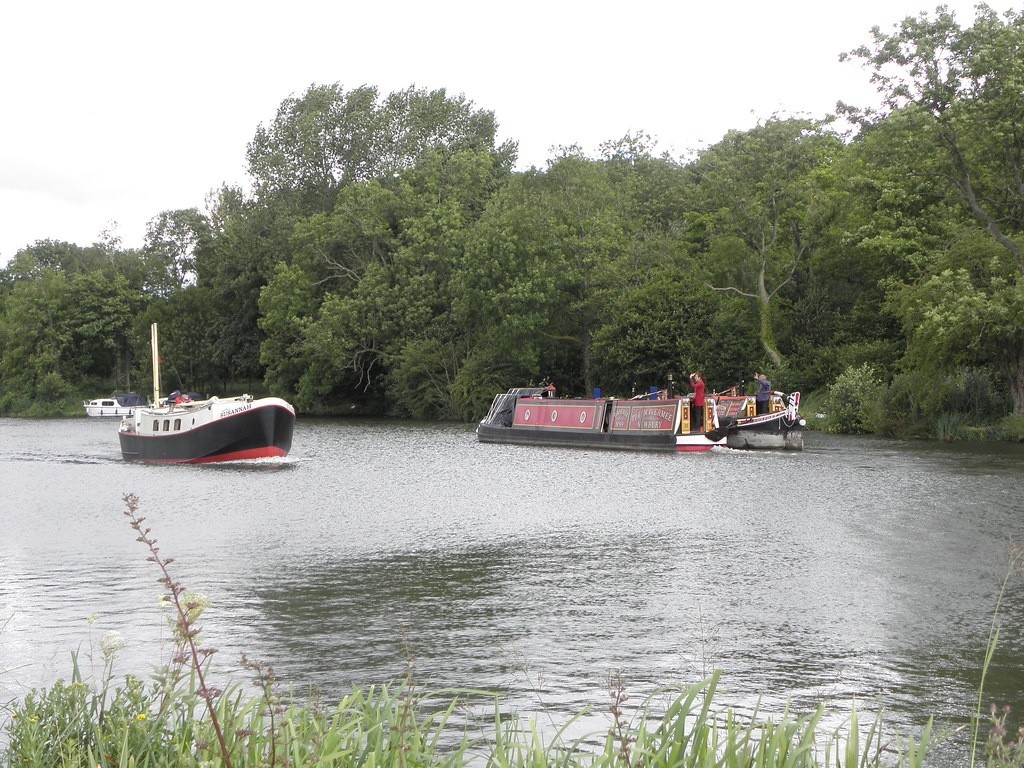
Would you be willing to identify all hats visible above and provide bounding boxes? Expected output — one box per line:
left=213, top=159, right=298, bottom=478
left=181, top=390, right=187, bottom=394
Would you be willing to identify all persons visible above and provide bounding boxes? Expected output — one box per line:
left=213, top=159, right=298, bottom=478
left=547, top=381, right=555, bottom=398
left=753, top=371, right=772, bottom=415
left=175, top=389, right=193, bottom=411
left=688, top=371, right=706, bottom=433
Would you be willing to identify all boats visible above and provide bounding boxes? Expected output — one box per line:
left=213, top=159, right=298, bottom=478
left=117, top=322, right=295, bottom=463
left=82, top=395, right=150, bottom=418
left=472, top=375, right=804, bottom=452
